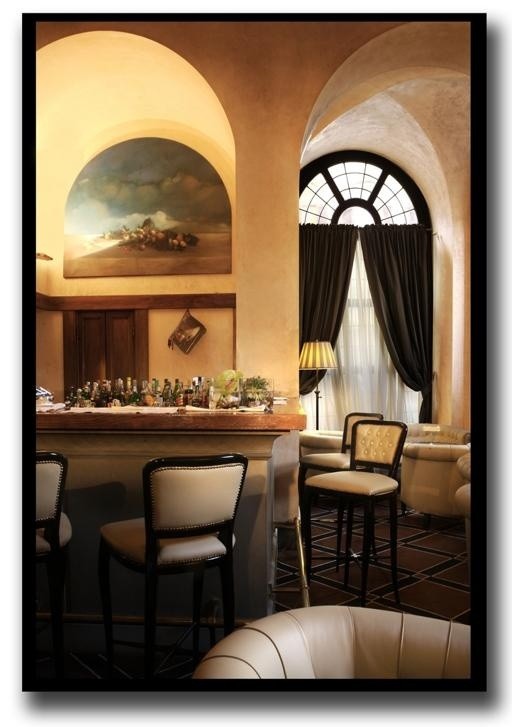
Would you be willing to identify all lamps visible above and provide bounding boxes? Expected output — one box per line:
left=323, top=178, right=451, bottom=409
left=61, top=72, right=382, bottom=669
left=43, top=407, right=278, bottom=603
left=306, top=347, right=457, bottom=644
left=299, top=340, right=338, bottom=430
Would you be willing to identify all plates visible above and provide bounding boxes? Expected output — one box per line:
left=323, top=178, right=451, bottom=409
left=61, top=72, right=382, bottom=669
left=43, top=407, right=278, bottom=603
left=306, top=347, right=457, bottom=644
left=240, top=406, right=266, bottom=411
left=274, top=397, right=288, bottom=401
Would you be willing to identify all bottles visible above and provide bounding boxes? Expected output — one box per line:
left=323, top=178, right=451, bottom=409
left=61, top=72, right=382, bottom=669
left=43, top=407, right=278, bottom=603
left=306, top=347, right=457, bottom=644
left=68, top=377, right=209, bottom=408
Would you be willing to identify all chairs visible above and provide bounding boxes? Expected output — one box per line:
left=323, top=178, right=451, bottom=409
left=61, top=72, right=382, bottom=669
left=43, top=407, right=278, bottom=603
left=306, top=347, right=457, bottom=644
left=306, top=420, right=408, bottom=604
left=193, top=605, right=471, bottom=679
left=35, top=450, right=72, bottom=676
left=301, top=413, right=384, bottom=572
left=97, top=453, right=248, bottom=683
left=299, top=430, right=343, bottom=506
left=400, top=443, right=471, bottom=529
